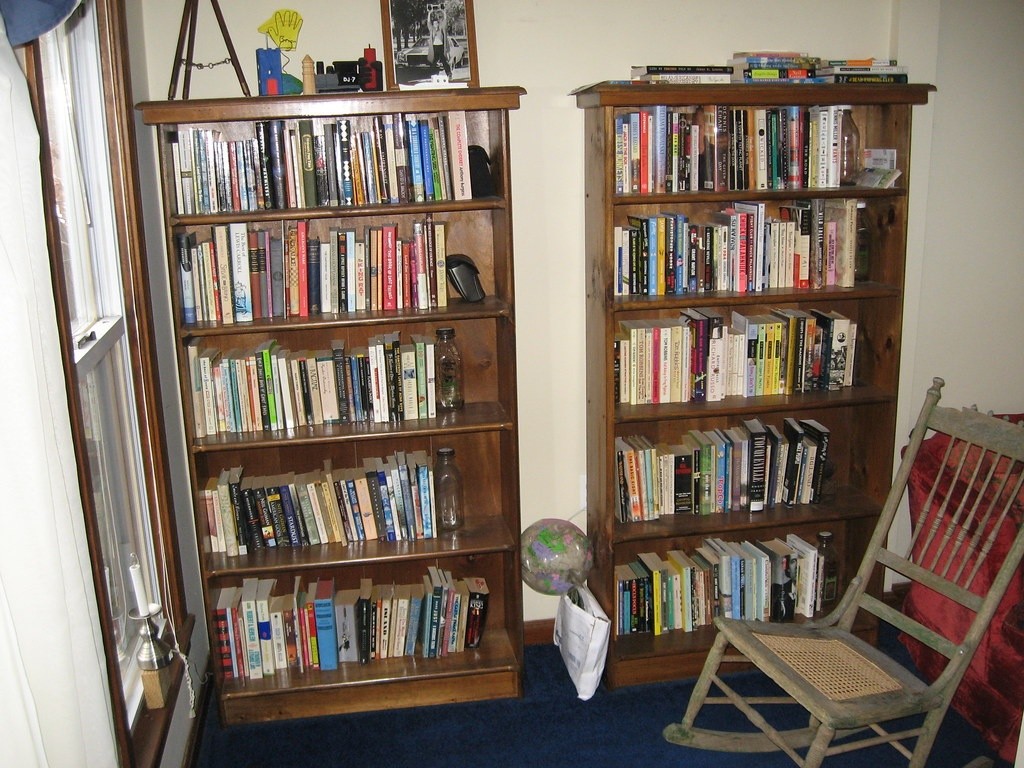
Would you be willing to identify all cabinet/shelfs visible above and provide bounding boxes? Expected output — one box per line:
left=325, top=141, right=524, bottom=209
left=135, top=85, right=530, bottom=733
left=574, top=79, right=938, bottom=684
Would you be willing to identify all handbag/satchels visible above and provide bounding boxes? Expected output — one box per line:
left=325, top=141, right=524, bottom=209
left=553, top=582, right=611, bottom=701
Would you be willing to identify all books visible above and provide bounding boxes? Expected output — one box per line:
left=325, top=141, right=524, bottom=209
left=162, top=111, right=489, bottom=682
left=611, top=49, right=910, bottom=639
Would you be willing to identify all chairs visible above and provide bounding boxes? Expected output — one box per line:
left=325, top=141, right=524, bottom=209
left=661, top=376, right=1024, bottom=768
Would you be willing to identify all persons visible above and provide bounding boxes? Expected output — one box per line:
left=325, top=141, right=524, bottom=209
left=426, top=6, right=453, bottom=84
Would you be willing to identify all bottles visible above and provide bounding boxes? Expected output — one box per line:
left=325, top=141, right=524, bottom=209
left=855, top=202, right=874, bottom=282
left=434, top=447, right=464, bottom=530
left=434, top=328, right=465, bottom=412
left=840, top=105, right=860, bottom=185
left=853, top=319, right=874, bottom=386
left=816, top=532, right=839, bottom=604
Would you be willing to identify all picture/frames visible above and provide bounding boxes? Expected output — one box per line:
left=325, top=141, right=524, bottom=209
left=380, top=0, right=480, bottom=91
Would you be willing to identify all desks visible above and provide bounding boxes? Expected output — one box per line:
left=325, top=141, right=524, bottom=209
left=901, top=415, right=1024, bottom=763
